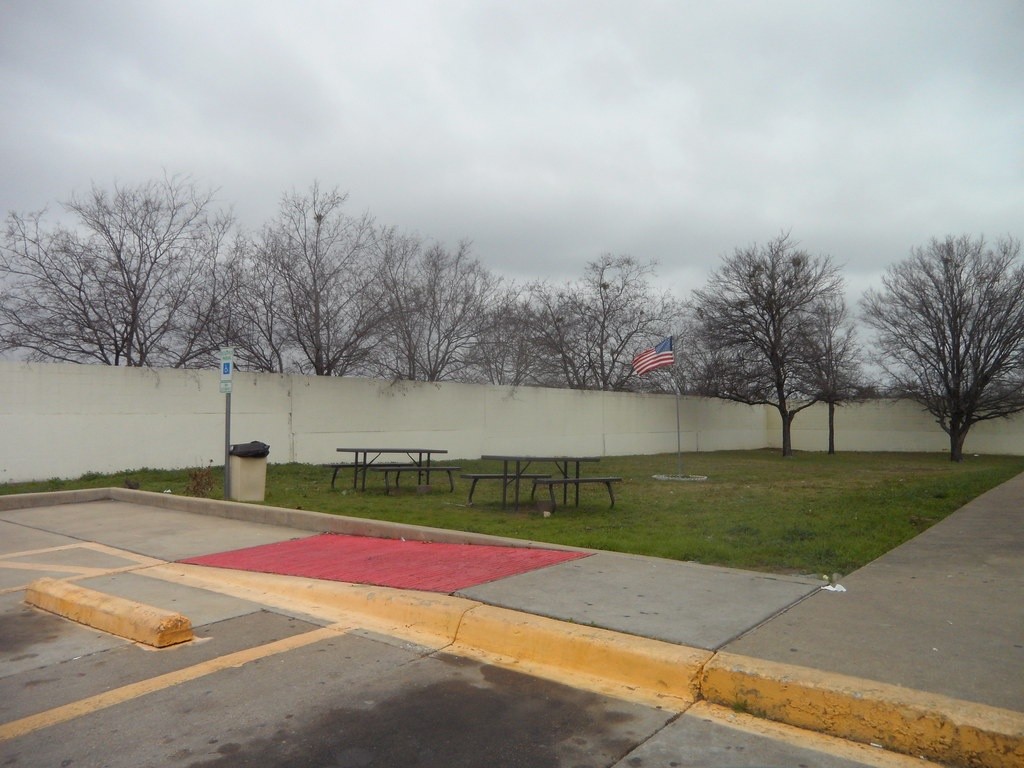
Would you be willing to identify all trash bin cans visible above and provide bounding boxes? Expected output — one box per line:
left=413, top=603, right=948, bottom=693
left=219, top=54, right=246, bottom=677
left=230, top=444, right=270, bottom=502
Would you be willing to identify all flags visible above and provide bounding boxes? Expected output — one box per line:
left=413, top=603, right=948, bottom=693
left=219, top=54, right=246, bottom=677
left=630, top=337, right=673, bottom=377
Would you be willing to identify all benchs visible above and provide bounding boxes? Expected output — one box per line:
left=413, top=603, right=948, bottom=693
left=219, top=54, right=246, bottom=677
left=325, top=463, right=415, bottom=492
left=459, top=473, right=551, bottom=508
left=482, top=455, right=601, bottom=509
left=532, top=477, right=621, bottom=515
left=372, top=465, right=461, bottom=496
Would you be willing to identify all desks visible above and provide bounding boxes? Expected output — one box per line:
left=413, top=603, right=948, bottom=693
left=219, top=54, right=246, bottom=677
left=336, top=445, right=449, bottom=493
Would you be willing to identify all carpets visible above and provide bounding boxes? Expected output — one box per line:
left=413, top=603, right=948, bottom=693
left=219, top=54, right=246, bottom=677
left=174, top=533, right=596, bottom=594
